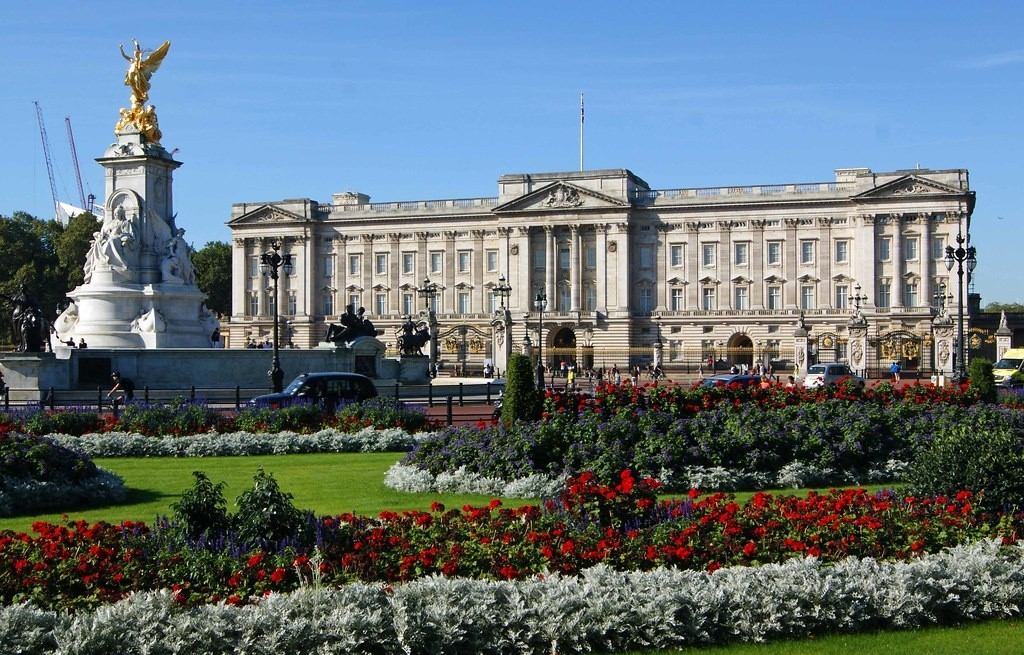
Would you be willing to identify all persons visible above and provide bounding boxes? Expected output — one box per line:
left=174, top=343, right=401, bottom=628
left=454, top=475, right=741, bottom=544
left=560, top=360, right=573, bottom=376
left=890, top=362, right=901, bottom=384
left=538, top=361, right=553, bottom=388
left=395, top=315, right=418, bottom=354
left=169, top=228, right=190, bottom=284
left=696, top=364, right=704, bottom=380
left=786, top=375, right=796, bottom=386
left=9, top=284, right=33, bottom=351
left=119, top=38, right=151, bottom=104
left=211, top=327, right=220, bottom=348
left=760, top=375, right=770, bottom=389
left=706, top=356, right=712, bottom=371
left=631, top=362, right=662, bottom=386
left=60, top=337, right=87, bottom=348
left=325, top=303, right=365, bottom=342
left=729, top=362, right=776, bottom=380
left=435, top=363, right=440, bottom=374
left=83, top=232, right=100, bottom=284
left=567, top=366, right=575, bottom=388
left=107, top=371, right=134, bottom=408
left=97, top=204, right=139, bottom=281
left=584, top=364, right=620, bottom=386
left=258, top=341, right=271, bottom=349
left=485, top=364, right=495, bottom=378
left=794, top=364, right=802, bottom=381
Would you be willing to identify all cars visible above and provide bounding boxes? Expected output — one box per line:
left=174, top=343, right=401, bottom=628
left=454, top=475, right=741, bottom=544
left=804, top=364, right=866, bottom=390
left=245, top=372, right=378, bottom=413
left=699, top=375, right=773, bottom=391
left=492, top=393, right=606, bottom=422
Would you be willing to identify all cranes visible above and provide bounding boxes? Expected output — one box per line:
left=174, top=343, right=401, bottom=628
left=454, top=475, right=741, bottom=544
left=33, top=100, right=62, bottom=224
left=65, top=115, right=96, bottom=211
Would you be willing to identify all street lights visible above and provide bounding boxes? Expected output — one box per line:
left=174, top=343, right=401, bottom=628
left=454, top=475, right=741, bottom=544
left=655, top=314, right=661, bottom=342
left=534, top=286, right=547, bottom=392
left=416, top=277, right=438, bottom=310
left=934, top=281, right=954, bottom=310
left=848, top=283, right=868, bottom=314
left=719, top=340, right=723, bottom=360
left=757, top=339, right=762, bottom=360
left=944, top=234, right=977, bottom=383
left=261, top=238, right=292, bottom=394
left=493, top=274, right=511, bottom=307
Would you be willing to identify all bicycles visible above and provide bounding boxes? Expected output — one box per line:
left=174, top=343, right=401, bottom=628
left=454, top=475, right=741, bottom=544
left=93, top=395, right=133, bottom=418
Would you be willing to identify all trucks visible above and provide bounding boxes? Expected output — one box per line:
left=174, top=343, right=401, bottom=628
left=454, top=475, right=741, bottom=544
left=991, top=348, right=1024, bottom=385
